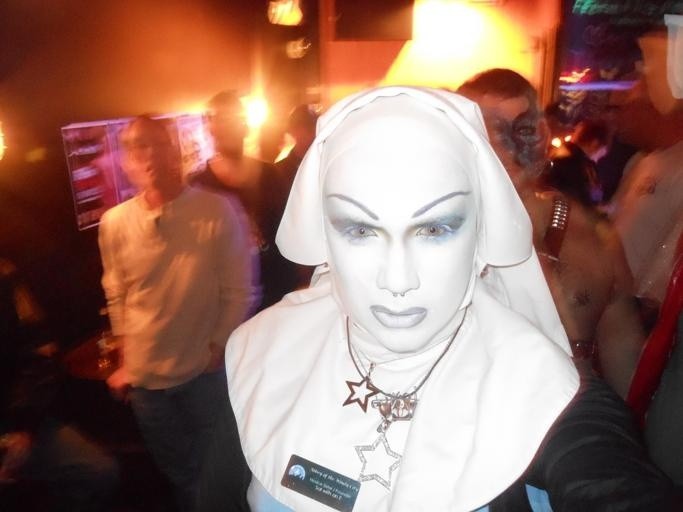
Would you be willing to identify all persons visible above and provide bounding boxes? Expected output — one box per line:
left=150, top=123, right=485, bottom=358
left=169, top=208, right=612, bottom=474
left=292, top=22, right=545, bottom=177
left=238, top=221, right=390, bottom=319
left=0, top=24, right=683, bottom=510
